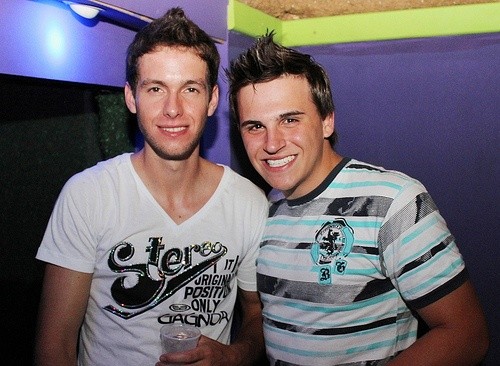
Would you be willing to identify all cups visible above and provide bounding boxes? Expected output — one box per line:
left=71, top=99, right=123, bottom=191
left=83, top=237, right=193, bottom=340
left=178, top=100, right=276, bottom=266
left=160, top=323, right=201, bottom=353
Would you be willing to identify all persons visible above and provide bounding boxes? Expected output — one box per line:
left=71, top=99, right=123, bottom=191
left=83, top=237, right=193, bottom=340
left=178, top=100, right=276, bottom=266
left=31, top=7, right=270, bottom=366
left=222, top=28, right=488, bottom=366
